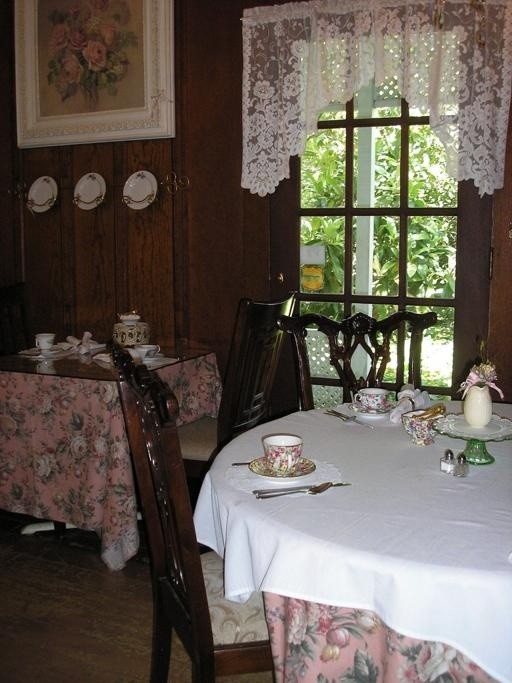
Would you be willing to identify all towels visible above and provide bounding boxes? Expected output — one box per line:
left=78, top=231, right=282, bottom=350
left=390, top=383, right=429, bottom=423
left=57, top=330, right=107, bottom=353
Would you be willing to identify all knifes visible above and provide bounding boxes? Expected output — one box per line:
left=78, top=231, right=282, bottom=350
left=326, top=410, right=375, bottom=430
left=253, top=482, right=352, bottom=495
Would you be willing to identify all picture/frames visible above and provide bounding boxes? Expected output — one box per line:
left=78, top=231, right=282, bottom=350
left=15, top=0, right=175, bottom=149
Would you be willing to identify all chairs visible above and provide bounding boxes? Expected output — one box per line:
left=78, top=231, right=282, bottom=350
left=272, top=312, right=437, bottom=412
left=176, top=291, right=302, bottom=462
left=104, top=340, right=274, bottom=681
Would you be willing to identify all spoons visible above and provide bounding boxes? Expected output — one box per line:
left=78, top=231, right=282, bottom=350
left=256, top=482, right=333, bottom=500
left=323, top=412, right=356, bottom=422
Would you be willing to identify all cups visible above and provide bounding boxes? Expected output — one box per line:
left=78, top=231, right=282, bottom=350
left=36, top=359, right=56, bottom=374
left=36, top=332, right=56, bottom=351
left=354, top=387, right=388, bottom=410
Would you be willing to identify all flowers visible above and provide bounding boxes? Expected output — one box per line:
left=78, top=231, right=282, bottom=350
left=41, top=0, right=136, bottom=107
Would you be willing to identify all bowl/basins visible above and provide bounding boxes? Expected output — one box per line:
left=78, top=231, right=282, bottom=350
left=262, top=432, right=304, bottom=475
left=401, top=409, right=444, bottom=447
left=135, top=342, right=159, bottom=359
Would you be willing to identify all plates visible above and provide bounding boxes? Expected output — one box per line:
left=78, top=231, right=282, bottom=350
left=28, top=175, right=58, bottom=213
left=348, top=402, right=393, bottom=416
left=18, top=345, right=79, bottom=359
left=73, top=172, right=107, bottom=210
left=248, top=456, right=316, bottom=479
left=123, top=170, right=158, bottom=210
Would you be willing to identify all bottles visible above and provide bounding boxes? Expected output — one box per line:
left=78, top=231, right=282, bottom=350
left=440, top=450, right=469, bottom=478
